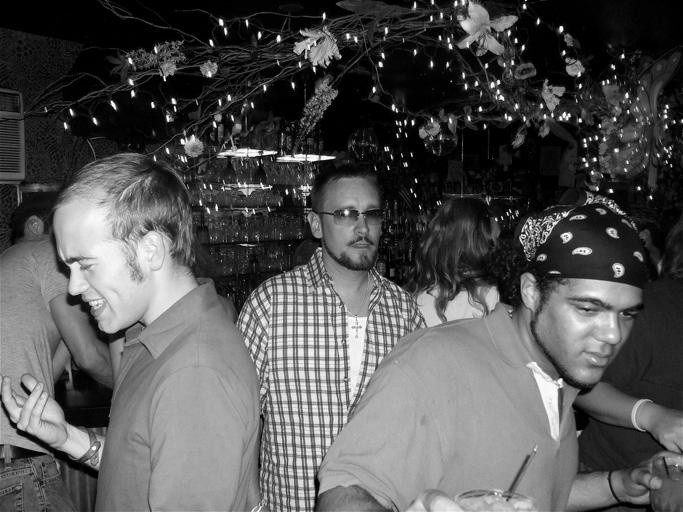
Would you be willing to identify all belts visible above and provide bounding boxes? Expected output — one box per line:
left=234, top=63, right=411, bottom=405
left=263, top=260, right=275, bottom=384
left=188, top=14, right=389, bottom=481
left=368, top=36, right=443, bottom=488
left=0, top=445, right=45, bottom=462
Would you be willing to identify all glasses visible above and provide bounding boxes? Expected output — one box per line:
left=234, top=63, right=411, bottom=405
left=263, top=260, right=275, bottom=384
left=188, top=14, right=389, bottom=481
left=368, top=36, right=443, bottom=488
left=314, top=208, right=388, bottom=226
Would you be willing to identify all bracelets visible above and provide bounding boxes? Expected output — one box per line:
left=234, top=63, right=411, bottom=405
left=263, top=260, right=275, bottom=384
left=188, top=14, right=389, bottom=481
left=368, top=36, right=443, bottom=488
left=630, top=397, right=654, bottom=433
left=607, top=470, right=622, bottom=504
left=65, top=424, right=100, bottom=467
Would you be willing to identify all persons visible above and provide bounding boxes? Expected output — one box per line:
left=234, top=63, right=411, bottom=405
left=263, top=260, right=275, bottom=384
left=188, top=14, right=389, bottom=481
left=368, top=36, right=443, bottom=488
left=312, top=201, right=682, bottom=511
left=414, top=196, right=502, bottom=328
left=231, top=162, right=426, bottom=511
left=569, top=211, right=683, bottom=474
left=0, top=152, right=270, bottom=511
left=0, top=191, right=113, bottom=512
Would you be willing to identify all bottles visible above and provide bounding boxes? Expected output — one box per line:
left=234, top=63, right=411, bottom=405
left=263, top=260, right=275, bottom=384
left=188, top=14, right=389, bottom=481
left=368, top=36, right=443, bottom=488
left=377, top=198, right=416, bottom=283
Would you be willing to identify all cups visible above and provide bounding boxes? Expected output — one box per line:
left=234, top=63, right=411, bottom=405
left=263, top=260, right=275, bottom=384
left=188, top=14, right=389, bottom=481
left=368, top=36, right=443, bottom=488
left=648, top=454, right=683, bottom=512
